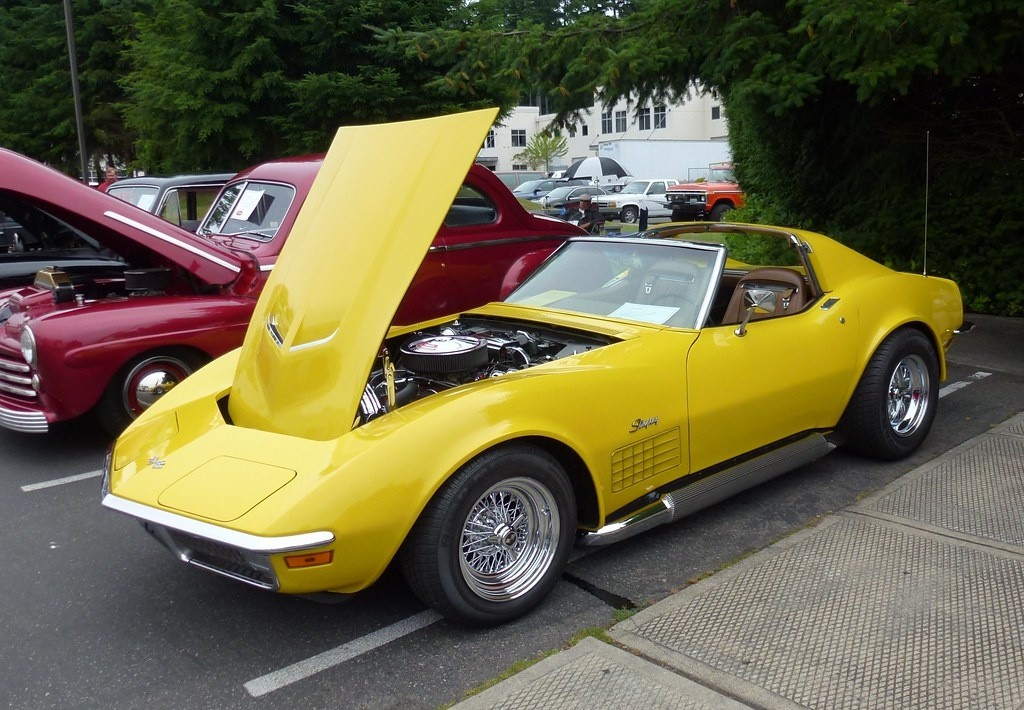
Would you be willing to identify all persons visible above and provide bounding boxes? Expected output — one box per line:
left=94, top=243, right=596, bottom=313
left=98, top=167, right=117, bottom=192
left=558, top=193, right=605, bottom=233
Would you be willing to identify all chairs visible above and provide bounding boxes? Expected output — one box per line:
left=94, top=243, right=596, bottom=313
left=632, top=260, right=700, bottom=322
left=568, top=211, right=604, bottom=236
left=724, top=267, right=805, bottom=327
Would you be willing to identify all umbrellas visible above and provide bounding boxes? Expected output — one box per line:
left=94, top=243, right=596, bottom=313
left=560, top=152, right=635, bottom=203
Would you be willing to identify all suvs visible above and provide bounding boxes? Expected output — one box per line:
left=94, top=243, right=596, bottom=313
left=663, top=161, right=745, bottom=225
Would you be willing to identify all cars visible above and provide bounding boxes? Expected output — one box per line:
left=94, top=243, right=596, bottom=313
left=492, top=170, right=593, bottom=201
left=530, top=184, right=615, bottom=221
left=589, top=179, right=681, bottom=225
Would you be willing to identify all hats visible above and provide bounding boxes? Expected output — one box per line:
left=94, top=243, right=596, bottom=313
left=579, top=194, right=592, bottom=201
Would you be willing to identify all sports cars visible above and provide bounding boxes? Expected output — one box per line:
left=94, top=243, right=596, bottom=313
left=0, top=143, right=617, bottom=442
left=99, top=105, right=978, bottom=633
